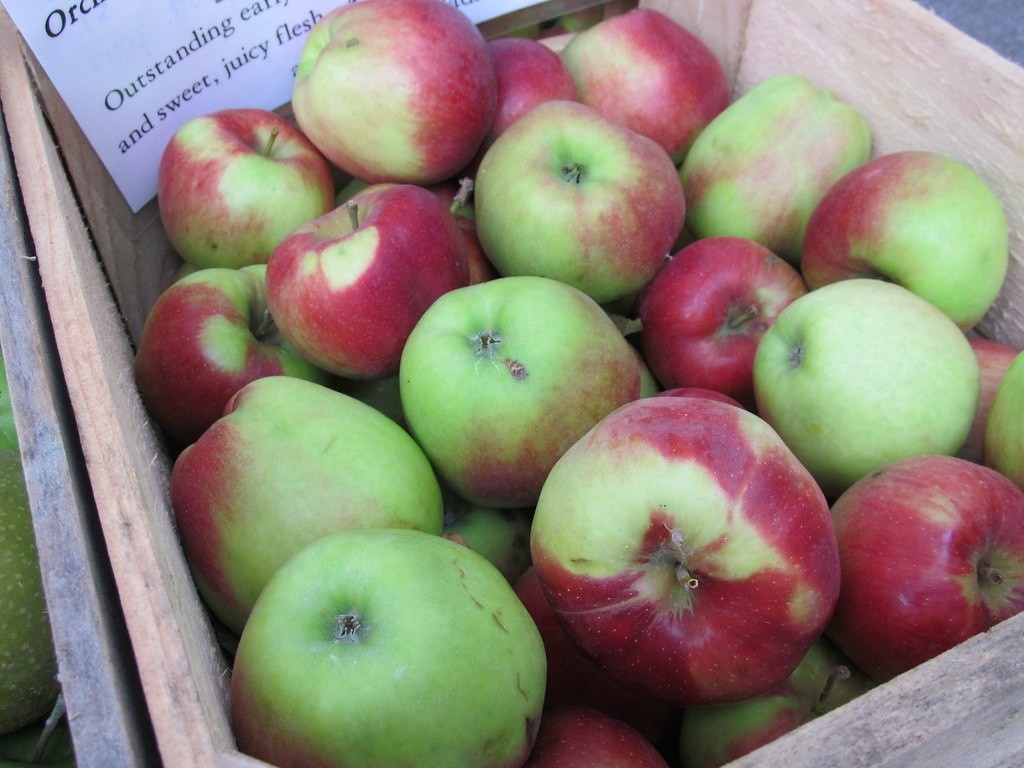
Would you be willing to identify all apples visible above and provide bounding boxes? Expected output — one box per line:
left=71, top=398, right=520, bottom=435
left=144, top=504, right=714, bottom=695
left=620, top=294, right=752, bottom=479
left=130, top=1, right=1024, bottom=768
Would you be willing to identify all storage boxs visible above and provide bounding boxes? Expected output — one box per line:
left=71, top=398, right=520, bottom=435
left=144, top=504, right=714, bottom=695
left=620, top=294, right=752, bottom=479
left=0, top=0, right=1024, bottom=768
